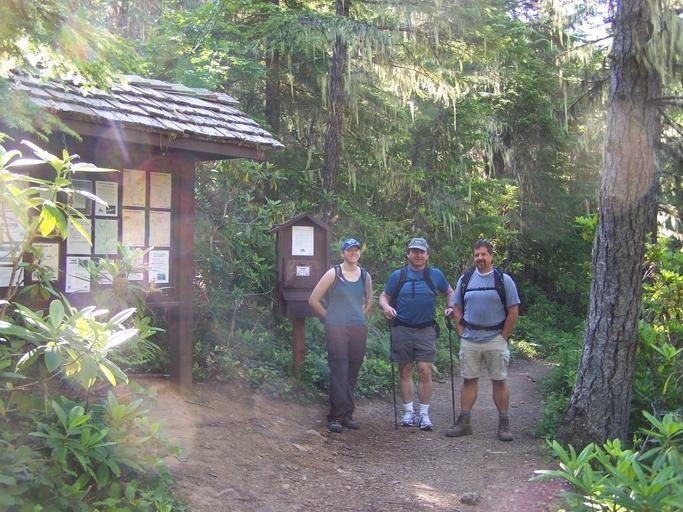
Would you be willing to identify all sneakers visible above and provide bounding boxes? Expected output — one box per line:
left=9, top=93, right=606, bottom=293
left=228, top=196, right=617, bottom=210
left=328, top=418, right=343, bottom=431
left=400, top=410, right=418, bottom=426
left=417, top=413, right=435, bottom=430
left=344, top=416, right=360, bottom=430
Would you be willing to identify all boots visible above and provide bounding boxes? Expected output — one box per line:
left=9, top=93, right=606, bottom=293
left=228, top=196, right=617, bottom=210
left=445, top=414, right=472, bottom=437
left=498, top=416, right=513, bottom=441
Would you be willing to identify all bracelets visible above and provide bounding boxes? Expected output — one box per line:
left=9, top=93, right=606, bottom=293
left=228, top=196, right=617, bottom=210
left=447, top=306, right=453, bottom=308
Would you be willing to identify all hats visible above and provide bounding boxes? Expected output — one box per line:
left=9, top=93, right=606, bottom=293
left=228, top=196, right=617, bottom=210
left=409, top=237, right=429, bottom=251
left=341, top=237, right=362, bottom=251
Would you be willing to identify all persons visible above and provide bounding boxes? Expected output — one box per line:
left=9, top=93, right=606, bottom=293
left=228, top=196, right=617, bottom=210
left=378, top=237, right=453, bottom=430
left=445, top=239, right=521, bottom=441
left=309, top=239, right=373, bottom=432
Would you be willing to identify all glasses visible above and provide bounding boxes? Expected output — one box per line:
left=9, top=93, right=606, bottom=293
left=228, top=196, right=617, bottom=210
left=472, top=238, right=491, bottom=247
